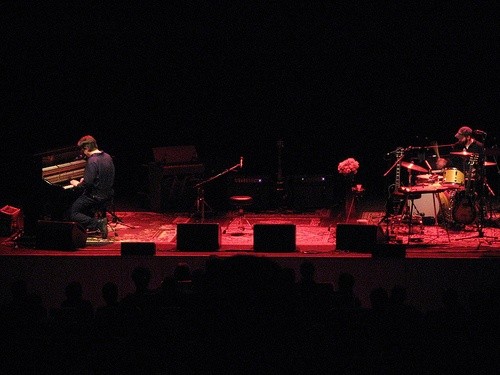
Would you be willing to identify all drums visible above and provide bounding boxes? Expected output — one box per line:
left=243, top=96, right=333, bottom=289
left=442, top=167, right=465, bottom=186
left=409, top=182, right=466, bottom=219
left=415, top=174, right=443, bottom=185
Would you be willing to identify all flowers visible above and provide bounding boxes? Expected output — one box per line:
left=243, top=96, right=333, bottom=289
left=337, top=157, right=360, bottom=176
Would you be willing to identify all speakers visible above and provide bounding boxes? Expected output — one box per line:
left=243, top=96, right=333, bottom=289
left=176, top=223, right=222, bottom=251
left=253, top=224, right=296, bottom=252
left=35, top=220, right=87, bottom=251
left=336, top=223, right=385, bottom=253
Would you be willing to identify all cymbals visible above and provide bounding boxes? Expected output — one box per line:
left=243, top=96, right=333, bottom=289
left=483, top=160, right=497, bottom=169
left=400, top=162, right=428, bottom=173
left=449, top=151, right=473, bottom=156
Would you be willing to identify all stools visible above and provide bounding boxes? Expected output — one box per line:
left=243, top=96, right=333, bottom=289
left=405, top=192, right=423, bottom=225
left=221, top=194, right=254, bottom=235
left=92, top=198, right=118, bottom=238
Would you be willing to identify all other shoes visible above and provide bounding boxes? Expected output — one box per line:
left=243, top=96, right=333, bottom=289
left=97, top=218, right=108, bottom=239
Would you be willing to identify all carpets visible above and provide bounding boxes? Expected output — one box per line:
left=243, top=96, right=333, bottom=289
left=2, top=211, right=194, bottom=245
left=221, top=212, right=500, bottom=247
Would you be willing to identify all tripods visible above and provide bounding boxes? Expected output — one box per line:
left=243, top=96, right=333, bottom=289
left=378, top=141, right=498, bottom=247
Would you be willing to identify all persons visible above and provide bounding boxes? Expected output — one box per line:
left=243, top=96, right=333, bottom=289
left=429, top=127, right=485, bottom=231
left=0, top=259, right=500, bottom=375
left=67, top=135, right=114, bottom=239
left=331, top=158, right=368, bottom=223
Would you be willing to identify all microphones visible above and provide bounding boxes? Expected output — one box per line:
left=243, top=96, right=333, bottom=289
left=240, top=157, right=243, bottom=168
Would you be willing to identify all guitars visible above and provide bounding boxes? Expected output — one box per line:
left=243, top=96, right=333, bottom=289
left=451, top=152, right=480, bottom=225
left=383, top=147, right=406, bottom=215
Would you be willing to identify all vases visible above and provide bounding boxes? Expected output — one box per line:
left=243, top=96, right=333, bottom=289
left=344, top=173, right=356, bottom=198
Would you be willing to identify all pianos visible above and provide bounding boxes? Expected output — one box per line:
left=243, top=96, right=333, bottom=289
left=30, top=145, right=108, bottom=222
left=143, top=143, right=207, bottom=213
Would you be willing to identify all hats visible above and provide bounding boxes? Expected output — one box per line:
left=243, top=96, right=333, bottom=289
left=455, top=126, right=471, bottom=138
